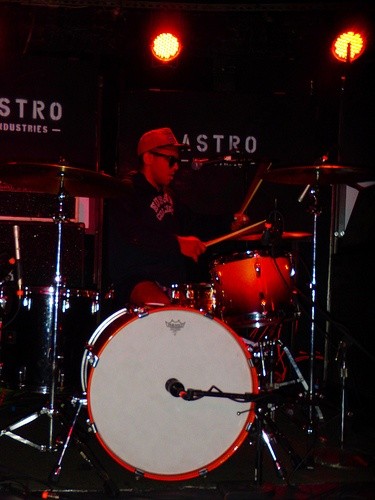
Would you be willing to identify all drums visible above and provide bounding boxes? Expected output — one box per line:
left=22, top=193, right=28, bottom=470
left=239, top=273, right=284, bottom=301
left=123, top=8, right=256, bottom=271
left=210, top=246, right=300, bottom=327
left=169, top=282, right=235, bottom=328
left=0, top=286, right=99, bottom=397
left=82, top=304, right=259, bottom=485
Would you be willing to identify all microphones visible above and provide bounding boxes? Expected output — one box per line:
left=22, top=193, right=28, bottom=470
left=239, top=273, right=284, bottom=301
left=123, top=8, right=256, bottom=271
left=164, top=378, right=194, bottom=401
left=190, top=155, right=225, bottom=170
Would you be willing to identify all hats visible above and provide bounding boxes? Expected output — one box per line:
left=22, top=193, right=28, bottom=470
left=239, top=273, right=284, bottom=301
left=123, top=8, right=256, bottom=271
left=137, top=127, right=190, bottom=157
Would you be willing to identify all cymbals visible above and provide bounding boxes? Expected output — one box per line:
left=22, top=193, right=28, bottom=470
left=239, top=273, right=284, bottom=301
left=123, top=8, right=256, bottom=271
left=1, top=160, right=135, bottom=199
left=236, top=230, right=312, bottom=239
left=265, top=164, right=374, bottom=186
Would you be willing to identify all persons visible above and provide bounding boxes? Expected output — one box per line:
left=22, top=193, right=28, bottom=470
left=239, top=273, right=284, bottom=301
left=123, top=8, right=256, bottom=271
left=114, top=129, right=204, bottom=312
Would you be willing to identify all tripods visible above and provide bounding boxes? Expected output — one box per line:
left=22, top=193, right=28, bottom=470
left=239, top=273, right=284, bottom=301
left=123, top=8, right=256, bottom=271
left=0, top=172, right=113, bottom=489
left=251, top=185, right=364, bottom=485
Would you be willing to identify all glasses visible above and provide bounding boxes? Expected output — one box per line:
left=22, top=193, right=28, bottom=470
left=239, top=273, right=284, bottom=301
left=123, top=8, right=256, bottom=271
left=148, top=150, right=182, bottom=170
